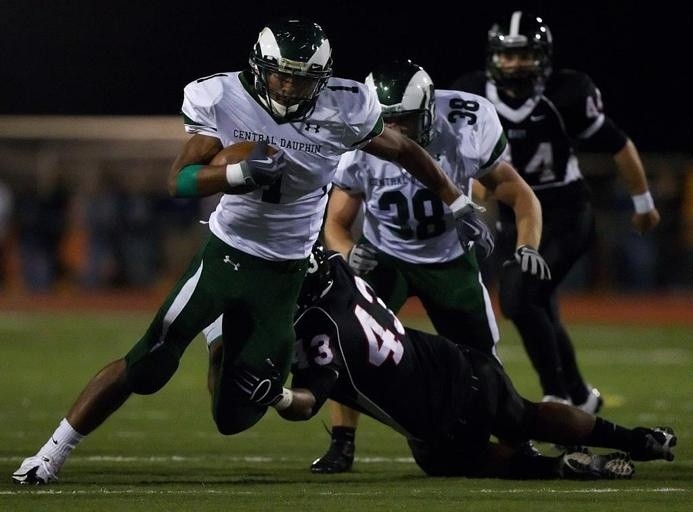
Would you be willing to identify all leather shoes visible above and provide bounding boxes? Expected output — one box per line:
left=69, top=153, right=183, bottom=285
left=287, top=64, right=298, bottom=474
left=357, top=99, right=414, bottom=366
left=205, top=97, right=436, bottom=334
left=310, top=432, right=354, bottom=473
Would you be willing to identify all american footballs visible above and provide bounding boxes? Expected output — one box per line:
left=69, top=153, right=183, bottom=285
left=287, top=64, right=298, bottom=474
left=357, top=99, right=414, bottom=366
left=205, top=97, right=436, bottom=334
left=210, top=142, right=279, bottom=194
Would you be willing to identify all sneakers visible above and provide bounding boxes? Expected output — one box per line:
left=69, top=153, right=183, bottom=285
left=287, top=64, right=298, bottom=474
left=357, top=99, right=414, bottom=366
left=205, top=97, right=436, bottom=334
left=12, top=456, right=49, bottom=486
left=635, top=426, right=677, bottom=461
left=556, top=446, right=635, bottom=480
left=540, top=388, right=603, bottom=416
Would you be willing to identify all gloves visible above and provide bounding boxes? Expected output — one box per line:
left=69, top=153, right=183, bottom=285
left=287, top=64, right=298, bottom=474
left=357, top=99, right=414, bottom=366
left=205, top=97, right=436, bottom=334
left=631, top=209, right=662, bottom=233
left=225, top=141, right=287, bottom=189
left=348, top=243, right=378, bottom=277
left=501, top=244, right=552, bottom=282
left=453, top=203, right=495, bottom=265
left=230, top=357, right=285, bottom=407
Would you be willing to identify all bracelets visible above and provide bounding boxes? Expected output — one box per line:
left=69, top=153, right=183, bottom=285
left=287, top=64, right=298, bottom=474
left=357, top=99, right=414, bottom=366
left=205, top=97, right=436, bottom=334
left=631, top=191, right=655, bottom=216
left=274, top=384, right=294, bottom=413
left=225, top=161, right=246, bottom=187
left=450, top=194, right=468, bottom=214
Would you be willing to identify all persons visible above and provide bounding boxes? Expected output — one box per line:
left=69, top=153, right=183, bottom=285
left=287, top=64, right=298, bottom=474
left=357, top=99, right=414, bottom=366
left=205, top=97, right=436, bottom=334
left=236, top=242, right=678, bottom=478
left=0, top=149, right=202, bottom=296
left=310, top=57, right=552, bottom=474
left=11, top=17, right=493, bottom=487
left=454, top=13, right=661, bottom=412
left=574, top=149, right=693, bottom=293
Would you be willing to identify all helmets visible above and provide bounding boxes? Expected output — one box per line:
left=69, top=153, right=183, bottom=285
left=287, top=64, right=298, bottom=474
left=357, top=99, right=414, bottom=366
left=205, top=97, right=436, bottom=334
left=485, top=12, right=552, bottom=94
left=364, top=60, right=435, bottom=149
left=247, top=19, right=333, bottom=125
